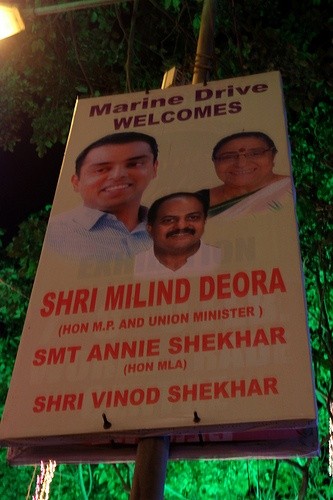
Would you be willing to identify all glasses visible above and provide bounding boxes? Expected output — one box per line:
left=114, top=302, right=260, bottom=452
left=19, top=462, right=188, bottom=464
left=214, top=146, right=274, bottom=162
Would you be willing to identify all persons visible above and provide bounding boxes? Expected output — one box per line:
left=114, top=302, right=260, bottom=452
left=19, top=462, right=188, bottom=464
left=46, top=132, right=159, bottom=257
left=134, top=192, right=219, bottom=276
left=197, top=132, right=293, bottom=215
